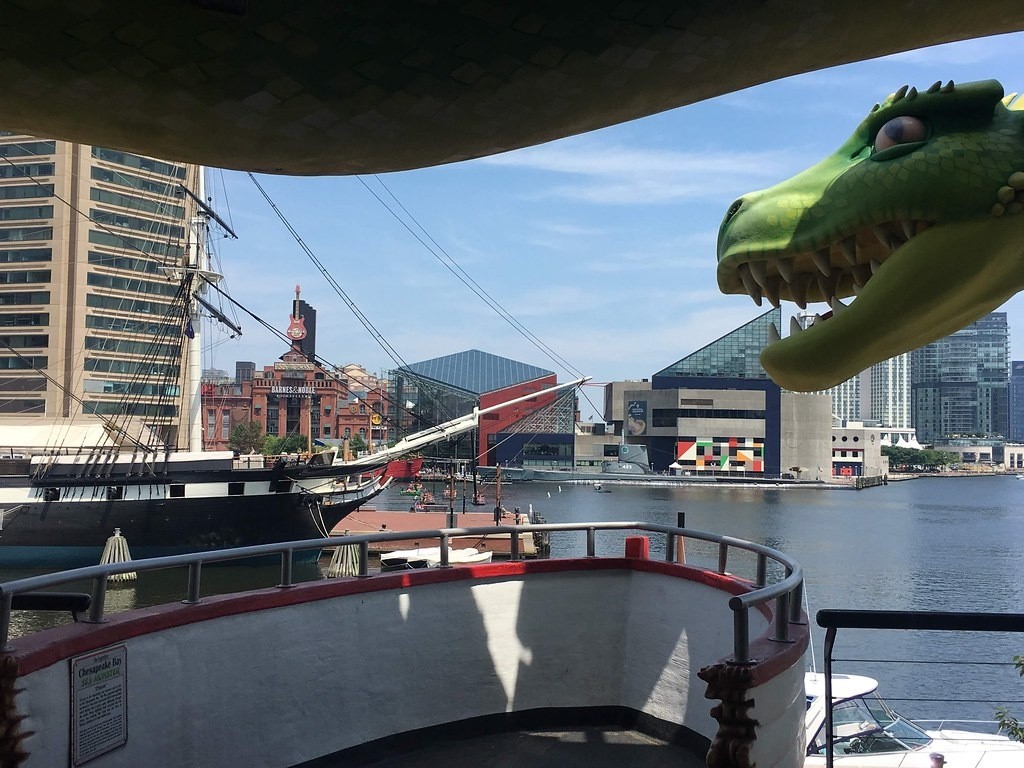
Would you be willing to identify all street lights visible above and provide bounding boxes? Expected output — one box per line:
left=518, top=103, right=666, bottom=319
left=303, top=396, right=313, bottom=459
left=367, top=404, right=373, bottom=452
left=380, top=368, right=389, bottom=446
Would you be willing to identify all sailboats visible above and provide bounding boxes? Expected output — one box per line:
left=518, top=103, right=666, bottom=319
left=0, top=132, right=593, bottom=572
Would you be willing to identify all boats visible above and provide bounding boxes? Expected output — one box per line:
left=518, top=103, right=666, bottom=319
left=1015, top=473, right=1024, bottom=479
left=399, top=479, right=485, bottom=513
left=593, top=483, right=612, bottom=493
left=798, top=576, right=1024, bottom=768
